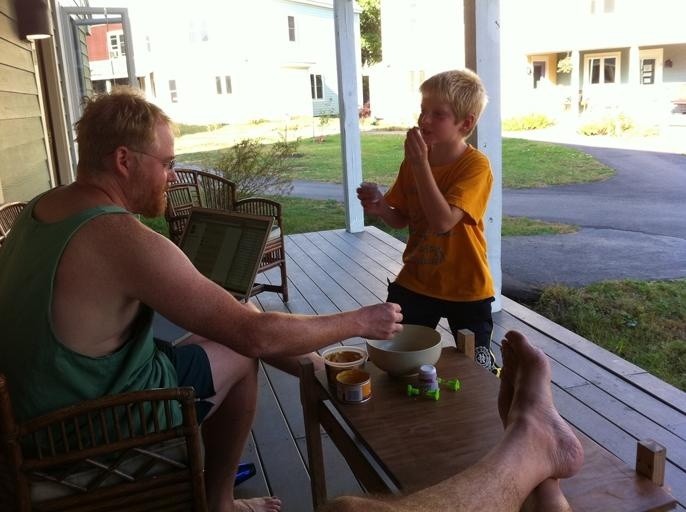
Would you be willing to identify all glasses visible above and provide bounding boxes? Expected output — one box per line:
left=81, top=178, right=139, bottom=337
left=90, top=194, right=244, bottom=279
left=104, top=146, right=176, bottom=170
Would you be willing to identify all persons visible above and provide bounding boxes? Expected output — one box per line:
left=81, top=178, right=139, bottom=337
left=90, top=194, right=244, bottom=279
left=357, top=67, right=496, bottom=364
left=0, top=87, right=404, bottom=512
left=315, top=329, right=584, bottom=512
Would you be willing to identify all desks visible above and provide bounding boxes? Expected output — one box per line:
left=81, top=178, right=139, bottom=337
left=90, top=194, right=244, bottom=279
left=296, top=329, right=681, bottom=511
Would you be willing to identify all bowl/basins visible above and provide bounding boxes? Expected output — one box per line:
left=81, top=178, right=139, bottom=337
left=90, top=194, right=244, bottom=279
left=365, top=324, right=442, bottom=378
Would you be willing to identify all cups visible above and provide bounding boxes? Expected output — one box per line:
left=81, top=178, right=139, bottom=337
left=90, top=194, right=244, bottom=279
left=322, top=345, right=369, bottom=388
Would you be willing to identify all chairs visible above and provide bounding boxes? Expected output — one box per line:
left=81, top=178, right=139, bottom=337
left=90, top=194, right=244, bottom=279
left=0, top=371, right=211, bottom=512
left=163, top=166, right=289, bottom=303
left=0, top=201, right=30, bottom=254
